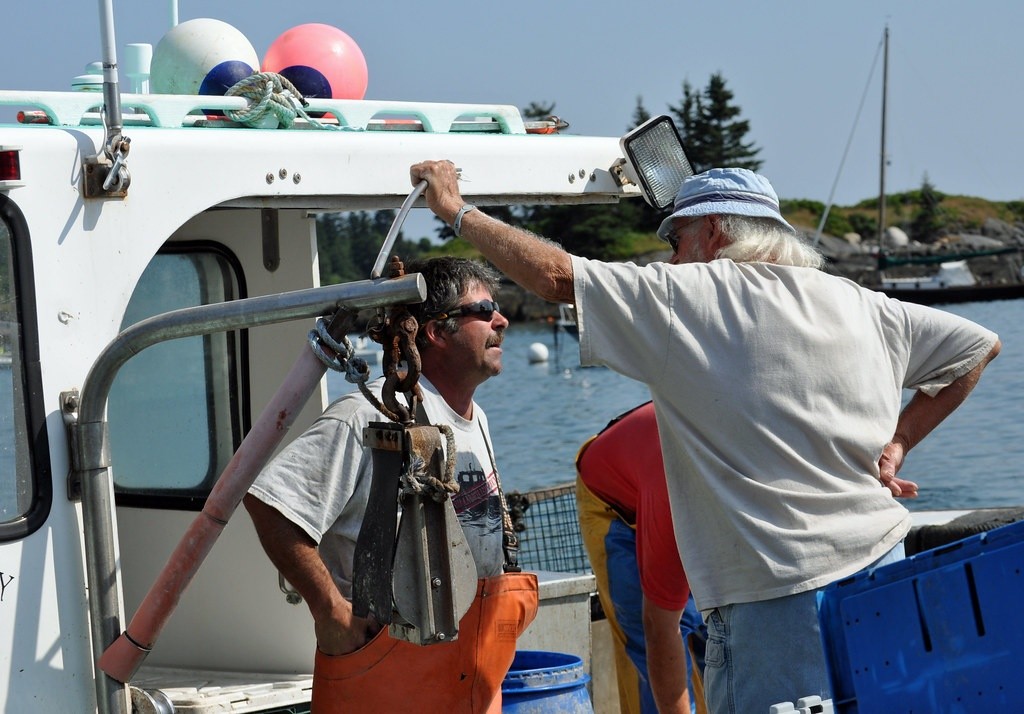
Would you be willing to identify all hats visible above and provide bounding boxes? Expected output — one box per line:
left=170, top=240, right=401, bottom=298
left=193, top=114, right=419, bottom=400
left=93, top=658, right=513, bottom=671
left=656, top=167, right=796, bottom=246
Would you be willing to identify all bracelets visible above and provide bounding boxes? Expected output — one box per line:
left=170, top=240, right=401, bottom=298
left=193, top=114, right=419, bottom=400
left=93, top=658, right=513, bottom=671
left=454, top=204, right=478, bottom=238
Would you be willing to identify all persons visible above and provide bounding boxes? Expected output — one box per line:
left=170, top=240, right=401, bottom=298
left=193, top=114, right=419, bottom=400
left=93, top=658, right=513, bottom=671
left=410, top=159, right=1002, bottom=714
left=573, top=399, right=708, bottom=714
left=243, top=256, right=539, bottom=714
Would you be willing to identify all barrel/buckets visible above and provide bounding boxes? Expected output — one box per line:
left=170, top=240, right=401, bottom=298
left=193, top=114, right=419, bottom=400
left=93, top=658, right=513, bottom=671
left=501, top=650, right=593, bottom=714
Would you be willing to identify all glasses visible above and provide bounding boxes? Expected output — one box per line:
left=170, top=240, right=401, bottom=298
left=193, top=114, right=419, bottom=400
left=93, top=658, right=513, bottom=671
left=665, top=217, right=706, bottom=254
left=419, top=299, right=500, bottom=323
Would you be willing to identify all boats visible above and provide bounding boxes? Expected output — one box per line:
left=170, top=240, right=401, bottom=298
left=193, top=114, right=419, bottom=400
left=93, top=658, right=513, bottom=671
left=1, top=0, right=1023, bottom=713
left=812, top=12, right=978, bottom=296
